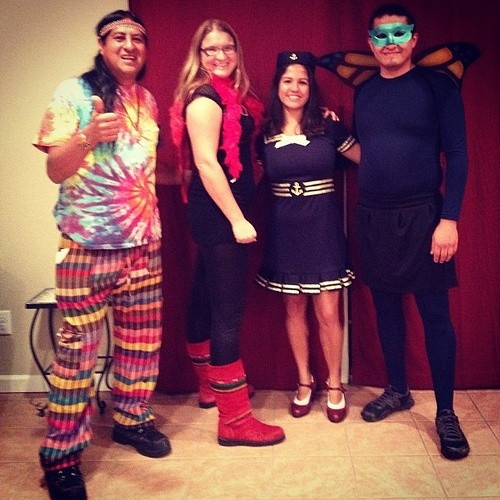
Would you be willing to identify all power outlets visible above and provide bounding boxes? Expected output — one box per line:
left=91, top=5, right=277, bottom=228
left=0, top=310, right=13, bottom=334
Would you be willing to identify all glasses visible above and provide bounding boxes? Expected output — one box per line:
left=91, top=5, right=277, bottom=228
left=199, top=45, right=236, bottom=56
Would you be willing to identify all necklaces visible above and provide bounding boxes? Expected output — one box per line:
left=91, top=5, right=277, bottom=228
left=115, top=87, right=140, bottom=138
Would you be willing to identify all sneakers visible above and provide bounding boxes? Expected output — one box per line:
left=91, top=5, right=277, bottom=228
left=111, top=423, right=170, bottom=458
left=435, top=409, right=470, bottom=459
left=44, top=465, right=88, bottom=500
left=361, top=384, right=415, bottom=422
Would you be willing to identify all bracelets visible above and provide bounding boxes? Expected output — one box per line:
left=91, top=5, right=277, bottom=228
left=78, top=133, right=92, bottom=150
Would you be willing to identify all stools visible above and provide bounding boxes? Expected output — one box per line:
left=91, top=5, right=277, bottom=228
left=24, top=287, right=114, bottom=418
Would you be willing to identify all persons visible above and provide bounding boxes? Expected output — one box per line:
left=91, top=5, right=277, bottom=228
left=170, top=18, right=339, bottom=446
left=337, top=5, right=470, bottom=462
left=254, top=50, right=361, bottom=424
left=29, top=9, right=171, bottom=500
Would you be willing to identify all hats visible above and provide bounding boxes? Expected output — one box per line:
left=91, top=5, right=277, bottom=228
left=277, top=52, right=318, bottom=68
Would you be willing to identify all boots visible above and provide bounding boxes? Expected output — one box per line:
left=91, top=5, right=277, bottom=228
left=206, top=358, right=285, bottom=446
left=184, top=338, right=255, bottom=409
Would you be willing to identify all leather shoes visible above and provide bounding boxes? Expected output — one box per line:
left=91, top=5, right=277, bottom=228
left=325, top=378, right=347, bottom=423
left=289, top=374, right=317, bottom=418
